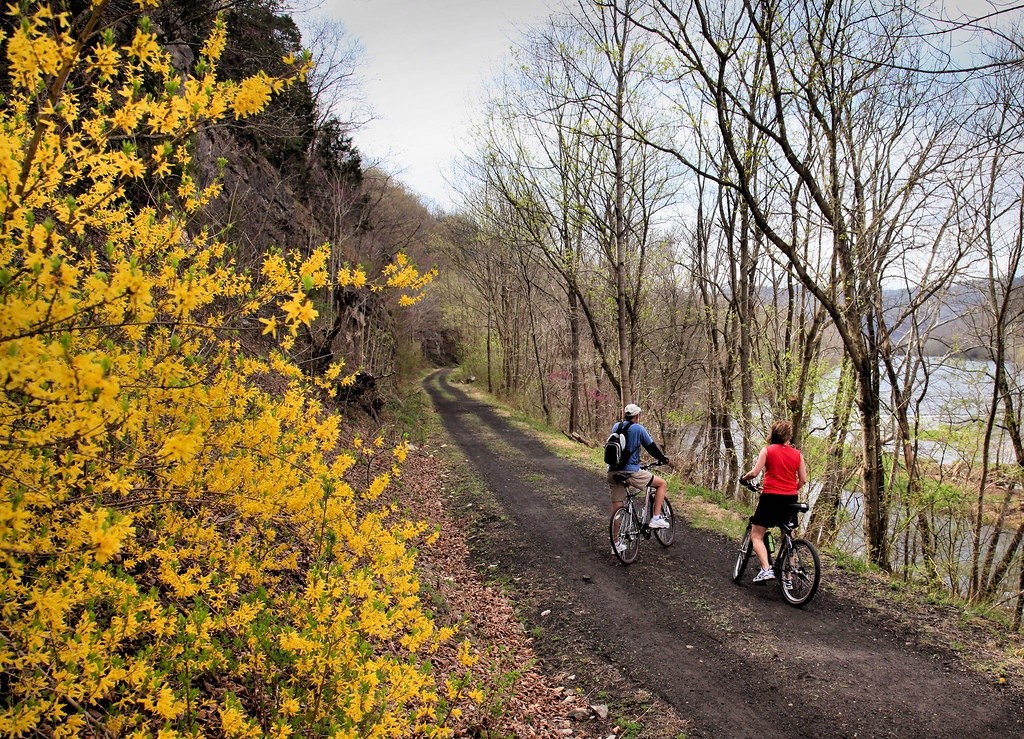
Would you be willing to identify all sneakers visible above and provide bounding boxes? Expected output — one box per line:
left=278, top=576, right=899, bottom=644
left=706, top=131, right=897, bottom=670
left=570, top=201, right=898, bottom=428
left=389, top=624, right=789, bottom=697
left=753, top=565, right=775, bottom=582
left=648, top=517, right=670, bottom=529
left=611, top=543, right=627, bottom=555
left=778, top=577, right=793, bottom=590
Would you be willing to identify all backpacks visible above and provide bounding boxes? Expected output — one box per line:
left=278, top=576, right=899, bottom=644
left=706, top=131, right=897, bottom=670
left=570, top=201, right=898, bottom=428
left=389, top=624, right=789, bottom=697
left=604, top=421, right=641, bottom=466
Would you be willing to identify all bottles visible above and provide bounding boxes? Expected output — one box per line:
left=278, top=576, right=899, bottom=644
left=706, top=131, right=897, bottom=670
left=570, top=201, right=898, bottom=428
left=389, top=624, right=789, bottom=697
left=768, top=532, right=775, bottom=552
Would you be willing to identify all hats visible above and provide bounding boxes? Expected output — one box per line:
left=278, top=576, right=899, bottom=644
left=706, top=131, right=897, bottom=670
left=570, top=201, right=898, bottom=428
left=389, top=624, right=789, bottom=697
left=625, top=404, right=641, bottom=417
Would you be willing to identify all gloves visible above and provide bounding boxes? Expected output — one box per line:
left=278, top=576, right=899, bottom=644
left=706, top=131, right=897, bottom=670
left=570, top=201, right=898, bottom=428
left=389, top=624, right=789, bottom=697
left=662, top=457, right=669, bottom=464
left=739, top=476, right=748, bottom=486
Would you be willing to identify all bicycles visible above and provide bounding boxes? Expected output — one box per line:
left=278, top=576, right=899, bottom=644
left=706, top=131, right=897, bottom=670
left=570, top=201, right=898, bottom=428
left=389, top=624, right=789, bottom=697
left=610, top=461, right=676, bottom=566
left=731, top=481, right=820, bottom=608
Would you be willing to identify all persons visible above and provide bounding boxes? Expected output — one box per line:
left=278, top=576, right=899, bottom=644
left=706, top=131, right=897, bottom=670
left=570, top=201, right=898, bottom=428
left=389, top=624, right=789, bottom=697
left=738, top=419, right=807, bottom=590
left=602, top=403, right=678, bottom=556
